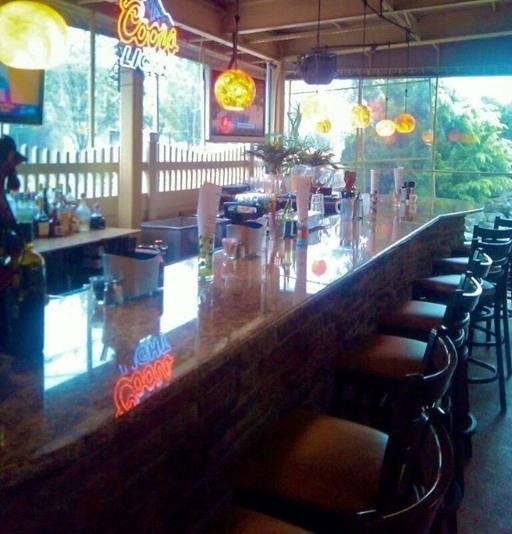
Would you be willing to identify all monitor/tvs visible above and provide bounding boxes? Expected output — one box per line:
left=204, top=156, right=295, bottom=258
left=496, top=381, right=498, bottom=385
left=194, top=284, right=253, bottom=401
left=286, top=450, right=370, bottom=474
left=205, top=64, right=267, bottom=144
left=0, top=60, right=45, bottom=125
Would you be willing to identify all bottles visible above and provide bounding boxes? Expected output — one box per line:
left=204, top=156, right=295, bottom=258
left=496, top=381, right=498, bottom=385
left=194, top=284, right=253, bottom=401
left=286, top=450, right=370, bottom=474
left=103, top=278, right=124, bottom=308
left=21, top=242, right=47, bottom=306
left=154, top=238, right=166, bottom=287
left=20, top=181, right=107, bottom=238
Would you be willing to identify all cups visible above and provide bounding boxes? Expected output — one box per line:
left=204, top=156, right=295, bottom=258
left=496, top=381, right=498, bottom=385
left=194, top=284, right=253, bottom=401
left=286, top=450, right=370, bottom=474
left=401, top=186, right=417, bottom=204
left=221, top=237, right=237, bottom=260
left=334, top=197, right=353, bottom=218
left=268, top=211, right=286, bottom=240
left=310, top=193, right=324, bottom=226
left=89, top=275, right=105, bottom=299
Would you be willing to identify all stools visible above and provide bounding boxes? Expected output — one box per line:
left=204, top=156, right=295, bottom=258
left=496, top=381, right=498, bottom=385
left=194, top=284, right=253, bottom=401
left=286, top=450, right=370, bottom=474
left=387, top=215, right=512, bottom=413
left=212, top=324, right=478, bottom=534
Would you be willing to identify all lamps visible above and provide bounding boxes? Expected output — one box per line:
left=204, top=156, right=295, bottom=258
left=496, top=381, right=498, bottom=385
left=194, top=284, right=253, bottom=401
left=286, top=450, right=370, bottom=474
left=310, top=2, right=417, bottom=138
left=213, top=0, right=258, bottom=112
left=0, top=1, right=71, bottom=73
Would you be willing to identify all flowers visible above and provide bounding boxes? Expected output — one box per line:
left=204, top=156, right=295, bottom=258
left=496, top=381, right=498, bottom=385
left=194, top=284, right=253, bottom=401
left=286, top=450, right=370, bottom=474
left=245, top=141, right=339, bottom=173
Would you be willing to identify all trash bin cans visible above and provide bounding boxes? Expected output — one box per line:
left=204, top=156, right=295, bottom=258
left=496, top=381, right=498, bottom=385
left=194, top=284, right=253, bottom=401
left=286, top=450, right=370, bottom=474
left=141, top=216, right=224, bottom=266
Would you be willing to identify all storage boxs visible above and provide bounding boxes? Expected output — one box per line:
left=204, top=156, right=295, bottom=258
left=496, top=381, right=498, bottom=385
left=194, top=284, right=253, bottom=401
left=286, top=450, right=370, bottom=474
left=141, top=215, right=231, bottom=260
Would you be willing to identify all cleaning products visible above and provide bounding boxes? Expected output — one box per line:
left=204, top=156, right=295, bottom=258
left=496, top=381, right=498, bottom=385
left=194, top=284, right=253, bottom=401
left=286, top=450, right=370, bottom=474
left=91, top=201, right=105, bottom=230
left=13, top=222, right=47, bottom=305
left=70, top=193, right=91, bottom=234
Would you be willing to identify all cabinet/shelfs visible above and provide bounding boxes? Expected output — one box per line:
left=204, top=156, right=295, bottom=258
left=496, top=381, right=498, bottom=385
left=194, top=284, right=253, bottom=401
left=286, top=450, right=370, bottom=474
left=30, top=226, right=142, bottom=296
